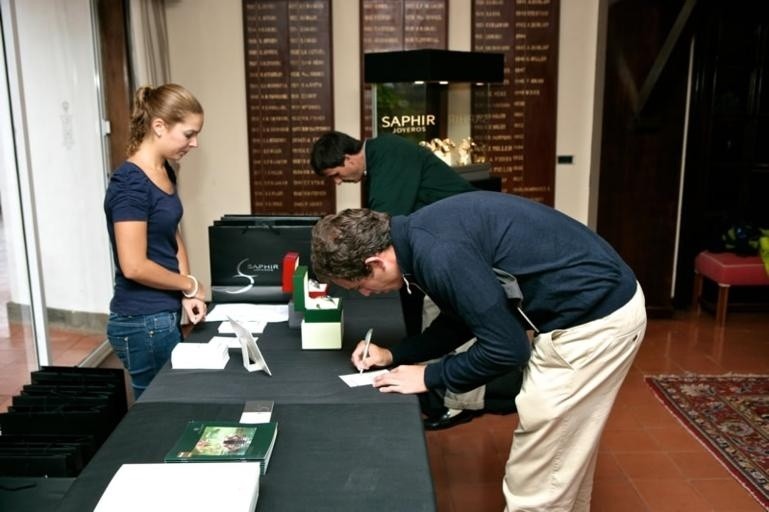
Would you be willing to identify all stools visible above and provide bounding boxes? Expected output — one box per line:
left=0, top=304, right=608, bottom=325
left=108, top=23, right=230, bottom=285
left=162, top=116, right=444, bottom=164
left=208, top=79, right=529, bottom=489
left=693, top=250, right=769, bottom=327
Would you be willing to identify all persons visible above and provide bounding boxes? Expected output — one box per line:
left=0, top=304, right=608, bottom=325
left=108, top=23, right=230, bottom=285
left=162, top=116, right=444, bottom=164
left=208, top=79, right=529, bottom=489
left=104, top=84, right=208, bottom=401
left=311, top=132, right=487, bottom=429
left=310, top=190, right=648, bottom=512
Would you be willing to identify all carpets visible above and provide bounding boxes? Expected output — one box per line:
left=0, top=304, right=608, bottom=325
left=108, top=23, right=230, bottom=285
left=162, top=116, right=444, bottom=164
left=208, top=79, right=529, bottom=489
left=645, top=372, right=769, bottom=507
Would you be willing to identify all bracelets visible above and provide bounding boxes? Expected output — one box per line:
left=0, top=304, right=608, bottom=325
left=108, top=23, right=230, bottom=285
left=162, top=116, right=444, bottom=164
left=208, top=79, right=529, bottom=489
left=182, top=274, right=198, bottom=298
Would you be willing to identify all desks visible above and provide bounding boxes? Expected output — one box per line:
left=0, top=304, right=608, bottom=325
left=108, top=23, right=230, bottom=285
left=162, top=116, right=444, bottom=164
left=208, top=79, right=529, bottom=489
left=58, top=301, right=434, bottom=512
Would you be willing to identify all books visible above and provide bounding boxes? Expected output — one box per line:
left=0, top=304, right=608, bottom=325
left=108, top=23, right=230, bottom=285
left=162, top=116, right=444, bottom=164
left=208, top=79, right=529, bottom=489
left=164, top=420, right=278, bottom=476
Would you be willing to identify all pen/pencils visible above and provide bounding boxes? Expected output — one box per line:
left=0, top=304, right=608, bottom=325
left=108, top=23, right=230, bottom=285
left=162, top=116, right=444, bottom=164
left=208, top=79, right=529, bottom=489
left=360, top=329, right=374, bottom=372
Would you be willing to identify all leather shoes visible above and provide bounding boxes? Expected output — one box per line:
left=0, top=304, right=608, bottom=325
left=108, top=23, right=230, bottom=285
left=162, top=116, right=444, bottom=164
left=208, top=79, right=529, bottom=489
left=424, top=410, right=472, bottom=429
